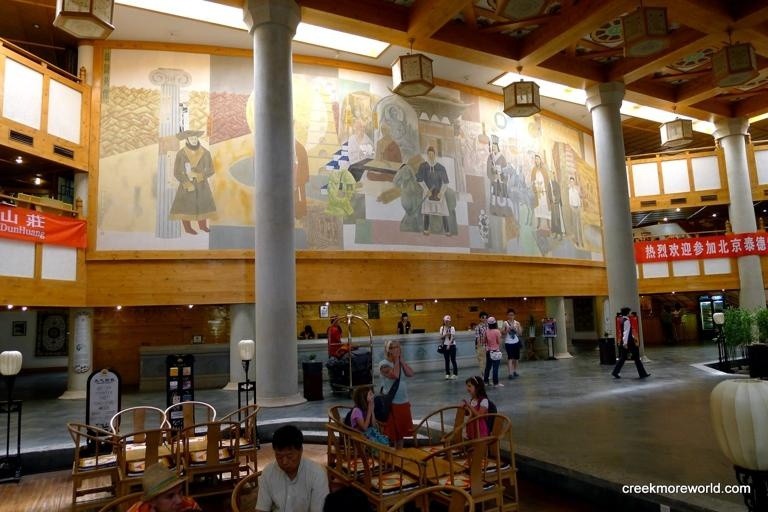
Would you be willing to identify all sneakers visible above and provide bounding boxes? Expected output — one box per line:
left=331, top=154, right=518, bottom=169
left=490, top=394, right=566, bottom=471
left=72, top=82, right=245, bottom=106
left=444, top=373, right=458, bottom=380
left=484, top=382, right=493, bottom=387
left=493, top=381, right=505, bottom=389
left=508, top=371, right=520, bottom=379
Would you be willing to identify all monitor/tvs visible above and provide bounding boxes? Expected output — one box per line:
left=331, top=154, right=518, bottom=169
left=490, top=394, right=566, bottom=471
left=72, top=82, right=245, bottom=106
left=413, top=329, right=425, bottom=333
left=318, top=334, right=328, bottom=339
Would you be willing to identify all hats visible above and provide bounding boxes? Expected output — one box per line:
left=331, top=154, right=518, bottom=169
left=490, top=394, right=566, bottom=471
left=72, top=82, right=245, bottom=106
left=138, top=460, right=191, bottom=502
left=487, top=316, right=497, bottom=325
left=443, top=315, right=452, bottom=322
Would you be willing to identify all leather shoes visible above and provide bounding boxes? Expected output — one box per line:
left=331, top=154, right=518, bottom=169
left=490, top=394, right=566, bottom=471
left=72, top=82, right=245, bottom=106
left=611, top=371, right=620, bottom=379
left=638, top=373, right=652, bottom=379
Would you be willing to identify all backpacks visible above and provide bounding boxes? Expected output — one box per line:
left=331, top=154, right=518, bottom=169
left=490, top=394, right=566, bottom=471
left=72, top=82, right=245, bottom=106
left=343, top=406, right=360, bottom=432
left=469, top=395, right=498, bottom=429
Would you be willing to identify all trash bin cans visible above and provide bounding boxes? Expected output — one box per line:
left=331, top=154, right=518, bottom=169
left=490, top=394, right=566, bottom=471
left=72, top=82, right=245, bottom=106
left=745, top=343, right=768, bottom=378
left=302, top=362, right=324, bottom=402
left=598, top=338, right=616, bottom=365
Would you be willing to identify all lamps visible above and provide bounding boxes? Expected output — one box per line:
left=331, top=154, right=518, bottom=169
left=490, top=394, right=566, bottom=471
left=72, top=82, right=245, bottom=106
left=713, top=312, right=728, bottom=365
left=391, top=37, right=435, bottom=97
left=711, top=26, right=760, bottom=88
left=658, top=106, right=694, bottom=148
left=53, top=0, right=115, bottom=40
left=502, top=66, right=541, bottom=117
left=620, top=0, right=672, bottom=57
left=0, top=351, right=23, bottom=484
left=238, top=340, right=260, bottom=450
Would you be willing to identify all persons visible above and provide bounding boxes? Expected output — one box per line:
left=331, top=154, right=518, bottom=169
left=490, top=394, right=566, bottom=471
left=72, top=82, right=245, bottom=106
left=396, top=311, right=412, bottom=334
left=168, top=130, right=216, bottom=235
left=611, top=307, right=652, bottom=380
left=474, top=309, right=523, bottom=387
left=126, top=460, right=202, bottom=512
left=375, top=338, right=416, bottom=447
left=326, top=314, right=343, bottom=359
left=461, top=375, right=498, bottom=439
left=343, top=386, right=376, bottom=432
left=294, top=120, right=584, bottom=247
left=254, top=424, right=330, bottom=512
left=300, top=325, right=315, bottom=339
left=437, top=314, right=459, bottom=380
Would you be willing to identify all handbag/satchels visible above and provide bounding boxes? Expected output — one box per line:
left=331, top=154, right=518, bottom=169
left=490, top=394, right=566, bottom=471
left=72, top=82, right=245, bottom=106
left=373, top=393, right=393, bottom=422
left=437, top=344, right=447, bottom=354
left=488, top=350, right=503, bottom=362
